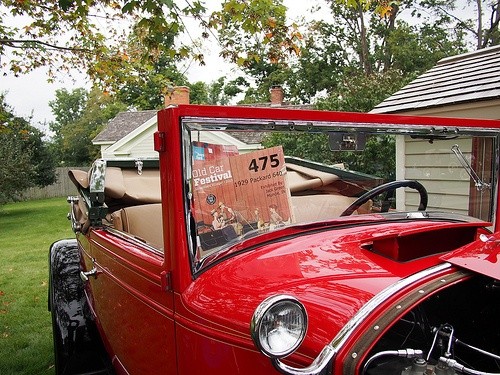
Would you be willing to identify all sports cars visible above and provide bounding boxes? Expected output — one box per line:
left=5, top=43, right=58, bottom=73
left=46, top=107, right=500, bottom=374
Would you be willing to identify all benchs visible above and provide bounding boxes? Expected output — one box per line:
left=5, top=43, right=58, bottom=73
left=108, top=189, right=373, bottom=250
